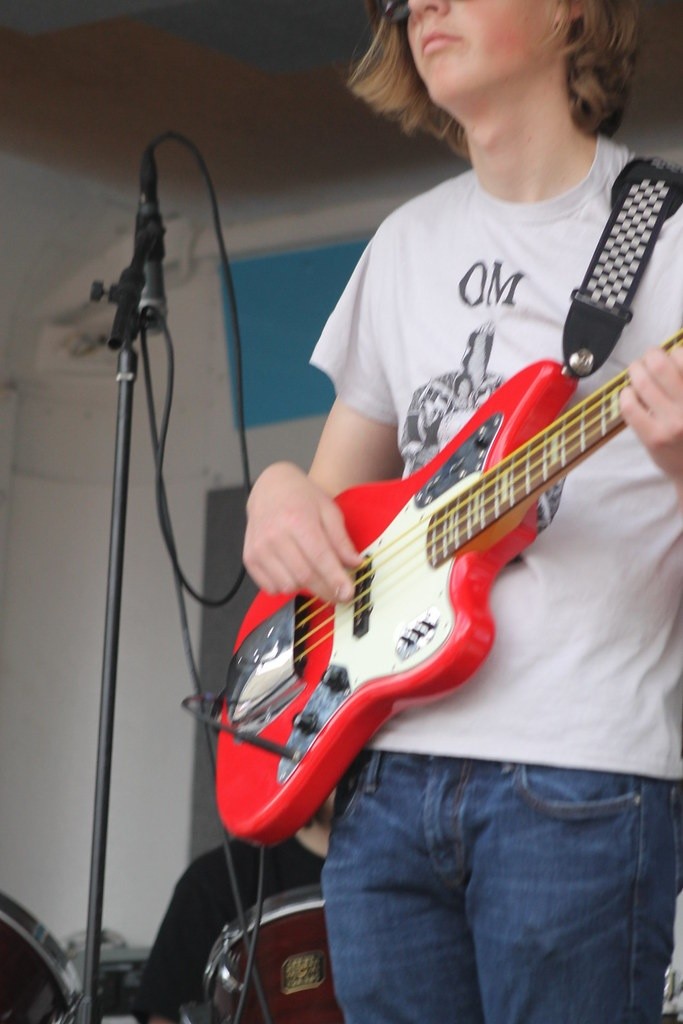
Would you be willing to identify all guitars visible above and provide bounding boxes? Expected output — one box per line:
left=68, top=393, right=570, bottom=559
left=209, top=320, right=683, bottom=847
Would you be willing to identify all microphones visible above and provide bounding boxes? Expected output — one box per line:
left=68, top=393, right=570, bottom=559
left=138, top=155, right=167, bottom=323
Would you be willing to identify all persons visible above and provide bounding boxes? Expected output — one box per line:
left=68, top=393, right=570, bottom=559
left=140, top=0, right=682, bottom=1024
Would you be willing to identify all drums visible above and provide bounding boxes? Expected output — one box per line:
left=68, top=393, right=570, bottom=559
left=201, top=881, right=345, bottom=1024
left=0, top=889, right=87, bottom=1023
